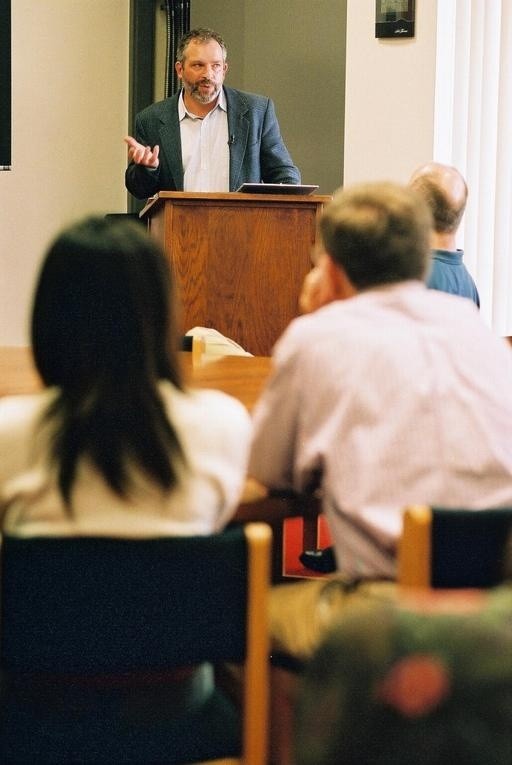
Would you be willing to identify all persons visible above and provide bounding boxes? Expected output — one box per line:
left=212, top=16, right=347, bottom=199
left=124, top=29, right=301, bottom=199
left=412, top=163, right=479, bottom=309
left=2, top=214, right=253, bottom=761
left=232, top=186, right=512, bottom=764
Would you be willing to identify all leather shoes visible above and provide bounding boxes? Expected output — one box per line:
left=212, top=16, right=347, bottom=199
left=300, top=546, right=336, bottom=573
